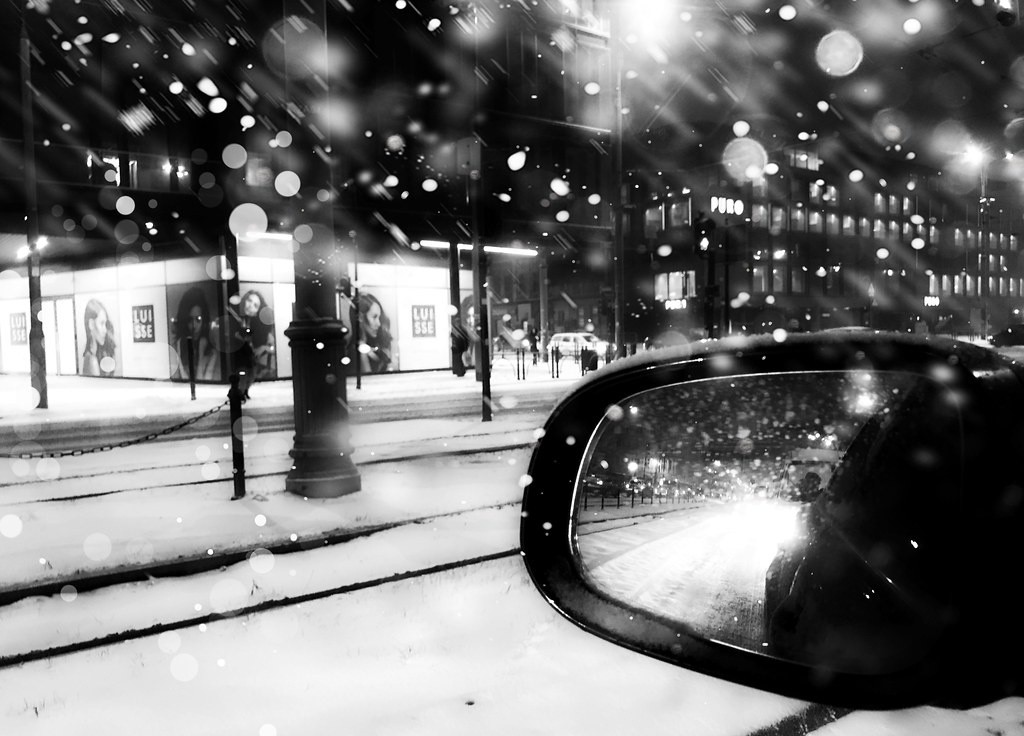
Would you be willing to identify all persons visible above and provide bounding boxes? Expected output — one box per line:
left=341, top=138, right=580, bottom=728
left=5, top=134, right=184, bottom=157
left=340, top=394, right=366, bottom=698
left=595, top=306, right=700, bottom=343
left=170, top=286, right=278, bottom=383
left=519, top=328, right=539, bottom=364
left=82, top=298, right=117, bottom=377
left=342, top=291, right=393, bottom=376
left=460, top=295, right=477, bottom=368
